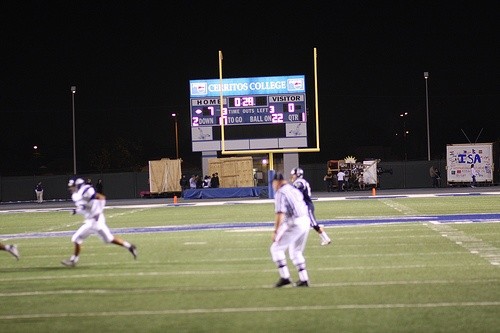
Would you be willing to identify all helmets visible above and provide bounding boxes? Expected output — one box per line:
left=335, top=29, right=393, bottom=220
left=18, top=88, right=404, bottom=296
left=68, top=176, right=85, bottom=186
left=291, top=167, right=303, bottom=178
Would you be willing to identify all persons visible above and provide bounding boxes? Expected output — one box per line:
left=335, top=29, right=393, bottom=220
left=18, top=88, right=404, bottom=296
left=471, top=164, right=477, bottom=188
left=290, top=168, right=331, bottom=245
left=180, top=172, right=220, bottom=198
left=0, top=242, right=20, bottom=260
left=337, top=169, right=346, bottom=192
left=60, top=177, right=137, bottom=267
left=35, top=181, right=44, bottom=204
left=269, top=173, right=310, bottom=288
left=324, top=171, right=334, bottom=192
left=430, top=166, right=439, bottom=188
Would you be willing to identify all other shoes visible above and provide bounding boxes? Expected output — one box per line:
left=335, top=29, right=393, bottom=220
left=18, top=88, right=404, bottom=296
left=129, top=244, right=137, bottom=259
left=8, top=244, right=20, bottom=261
left=321, top=239, right=330, bottom=245
left=292, top=279, right=310, bottom=287
left=61, top=259, right=75, bottom=267
left=275, top=277, right=291, bottom=287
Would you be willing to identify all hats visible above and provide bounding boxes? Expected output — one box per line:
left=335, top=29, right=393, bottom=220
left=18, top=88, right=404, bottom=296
left=269, top=174, right=284, bottom=182
